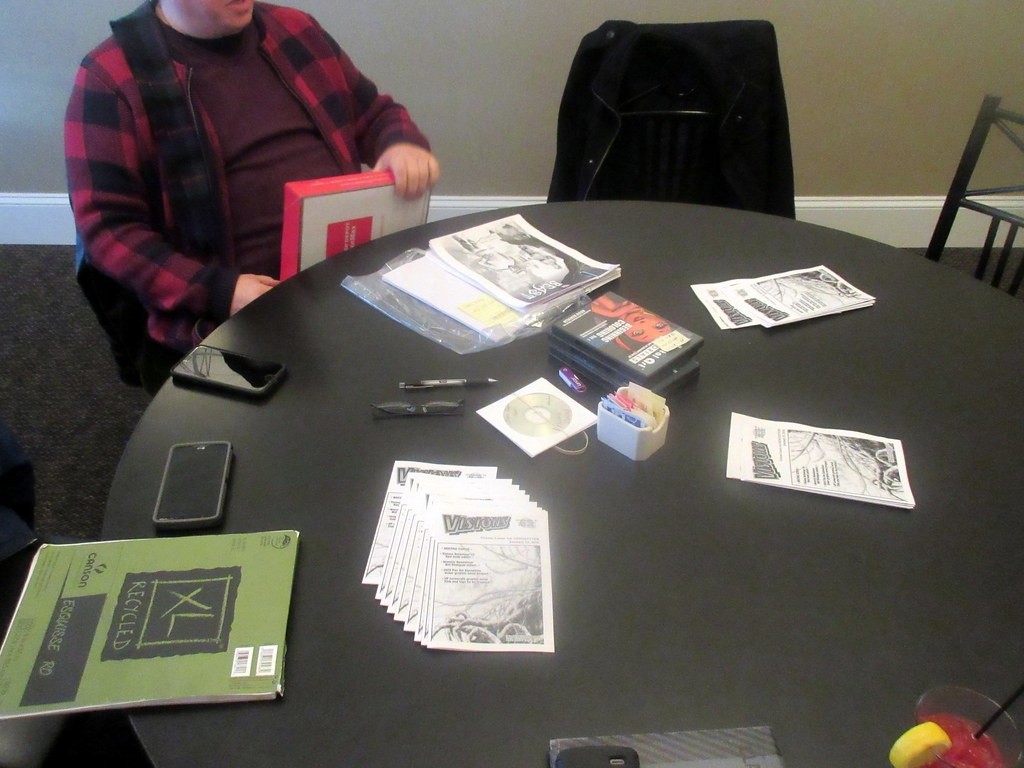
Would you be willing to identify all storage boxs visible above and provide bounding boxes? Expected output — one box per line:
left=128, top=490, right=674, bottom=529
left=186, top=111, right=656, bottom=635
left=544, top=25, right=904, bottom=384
left=543, top=290, right=704, bottom=393
left=278, top=169, right=431, bottom=278
left=597, top=387, right=670, bottom=460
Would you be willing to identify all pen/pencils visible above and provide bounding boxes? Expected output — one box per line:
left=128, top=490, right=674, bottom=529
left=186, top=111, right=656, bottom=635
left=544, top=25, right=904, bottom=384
left=397, top=376, right=498, bottom=389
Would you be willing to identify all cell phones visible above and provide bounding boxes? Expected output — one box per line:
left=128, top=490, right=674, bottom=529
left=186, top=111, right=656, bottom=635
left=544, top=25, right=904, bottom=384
left=170, top=344, right=287, bottom=399
left=153, top=440, right=235, bottom=529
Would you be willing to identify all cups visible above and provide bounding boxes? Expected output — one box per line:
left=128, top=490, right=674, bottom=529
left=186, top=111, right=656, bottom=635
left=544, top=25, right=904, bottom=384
left=915, top=685, right=1024, bottom=768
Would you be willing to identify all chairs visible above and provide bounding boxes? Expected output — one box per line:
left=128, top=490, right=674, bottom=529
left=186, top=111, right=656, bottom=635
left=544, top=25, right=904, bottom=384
left=79, top=257, right=180, bottom=396
left=547, top=14, right=796, bottom=218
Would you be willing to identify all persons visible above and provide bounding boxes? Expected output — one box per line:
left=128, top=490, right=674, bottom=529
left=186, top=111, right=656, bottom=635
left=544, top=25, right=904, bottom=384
left=64, top=1, right=441, bottom=344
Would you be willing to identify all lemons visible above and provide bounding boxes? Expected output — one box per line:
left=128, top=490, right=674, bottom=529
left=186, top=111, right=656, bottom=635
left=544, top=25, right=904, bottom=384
left=889, top=722, right=953, bottom=768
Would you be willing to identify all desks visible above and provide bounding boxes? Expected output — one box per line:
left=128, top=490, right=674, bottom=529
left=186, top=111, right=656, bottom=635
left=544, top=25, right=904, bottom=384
left=96, top=197, right=1024, bottom=768
